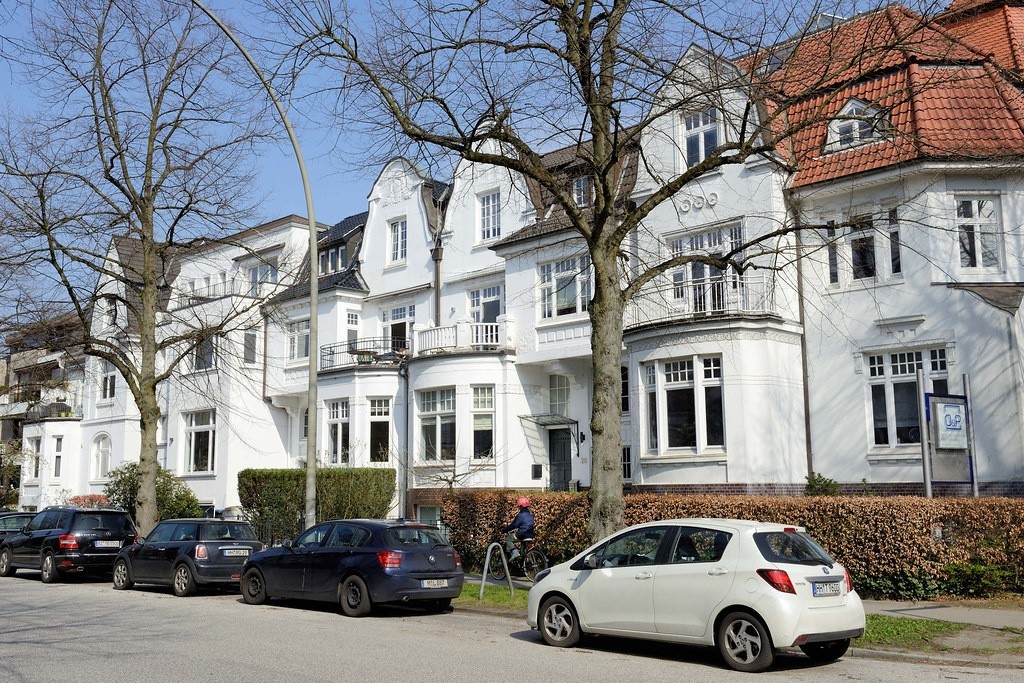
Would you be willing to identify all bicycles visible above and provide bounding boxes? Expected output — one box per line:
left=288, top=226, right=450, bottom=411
left=489, top=523, right=550, bottom=584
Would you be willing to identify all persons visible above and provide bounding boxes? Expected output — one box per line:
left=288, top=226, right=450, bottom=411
left=501, top=496, right=535, bottom=563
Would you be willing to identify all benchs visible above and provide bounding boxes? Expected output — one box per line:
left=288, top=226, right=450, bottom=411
left=372, top=348, right=410, bottom=365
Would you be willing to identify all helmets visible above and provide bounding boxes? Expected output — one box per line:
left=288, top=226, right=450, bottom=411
left=516, top=498, right=530, bottom=509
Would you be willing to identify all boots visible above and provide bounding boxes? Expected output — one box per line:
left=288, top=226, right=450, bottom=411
left=509, top=549, right=520, bottom=562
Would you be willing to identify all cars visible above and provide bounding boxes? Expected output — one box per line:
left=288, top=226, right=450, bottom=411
left=112, top=516, right=266, bottom=596
left=240, top=518, right=466, bottom=616
left=0, top=511, right=40, bottom=547
left=0, top=504, right=139, bottom=583
left=526, top=517, right=867, bottom=673
